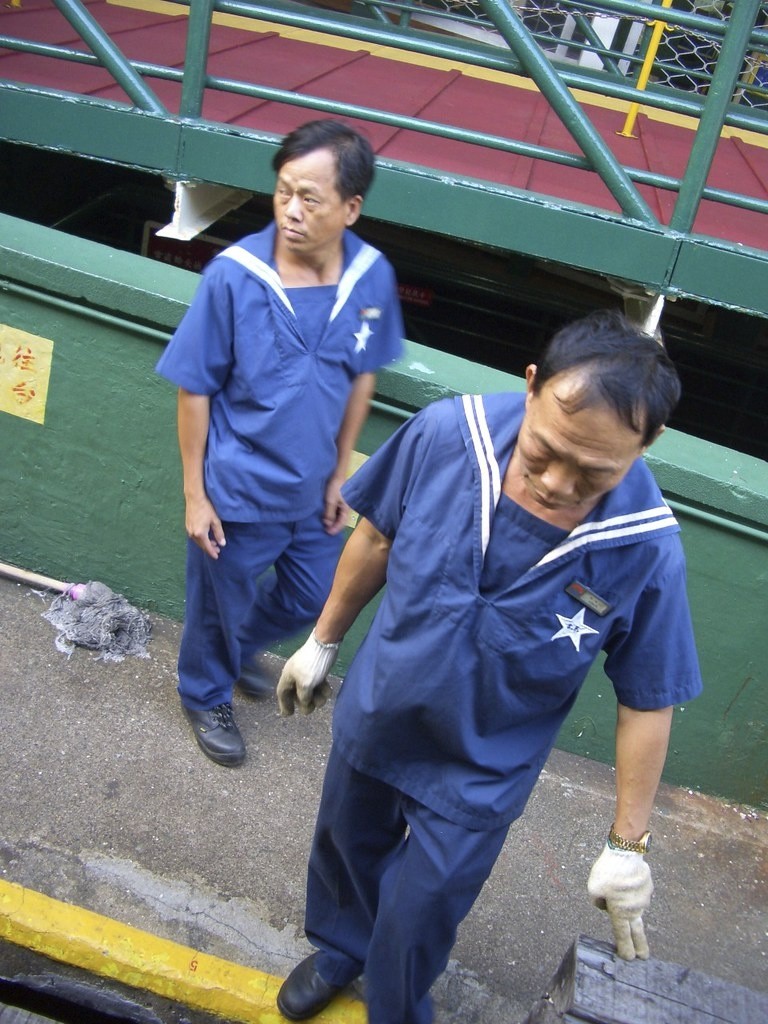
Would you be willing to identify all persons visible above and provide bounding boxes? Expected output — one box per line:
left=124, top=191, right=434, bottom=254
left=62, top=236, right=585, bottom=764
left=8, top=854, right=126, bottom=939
left=152, top=117, right=405, bottom=772
left=270, top=304, right=701, bottom=1023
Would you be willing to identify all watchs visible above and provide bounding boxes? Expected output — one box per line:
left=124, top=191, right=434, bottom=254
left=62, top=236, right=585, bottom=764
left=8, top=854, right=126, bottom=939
left=606, top=824, right=652, bottom=854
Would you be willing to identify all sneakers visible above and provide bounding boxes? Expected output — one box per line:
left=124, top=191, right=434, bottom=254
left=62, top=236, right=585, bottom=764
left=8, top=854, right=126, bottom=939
left=276, top=947, right=341, bottom=1021
left=180, top=702, right=246, bottom=766
left=238, top=661, right=270, bottom=696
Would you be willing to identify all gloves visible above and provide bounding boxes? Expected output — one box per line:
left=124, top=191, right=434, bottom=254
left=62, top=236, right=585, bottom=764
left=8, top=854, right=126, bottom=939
left=273, top=627, right=344, bottom=716
left=587, top=840, right=655, bottom=962
